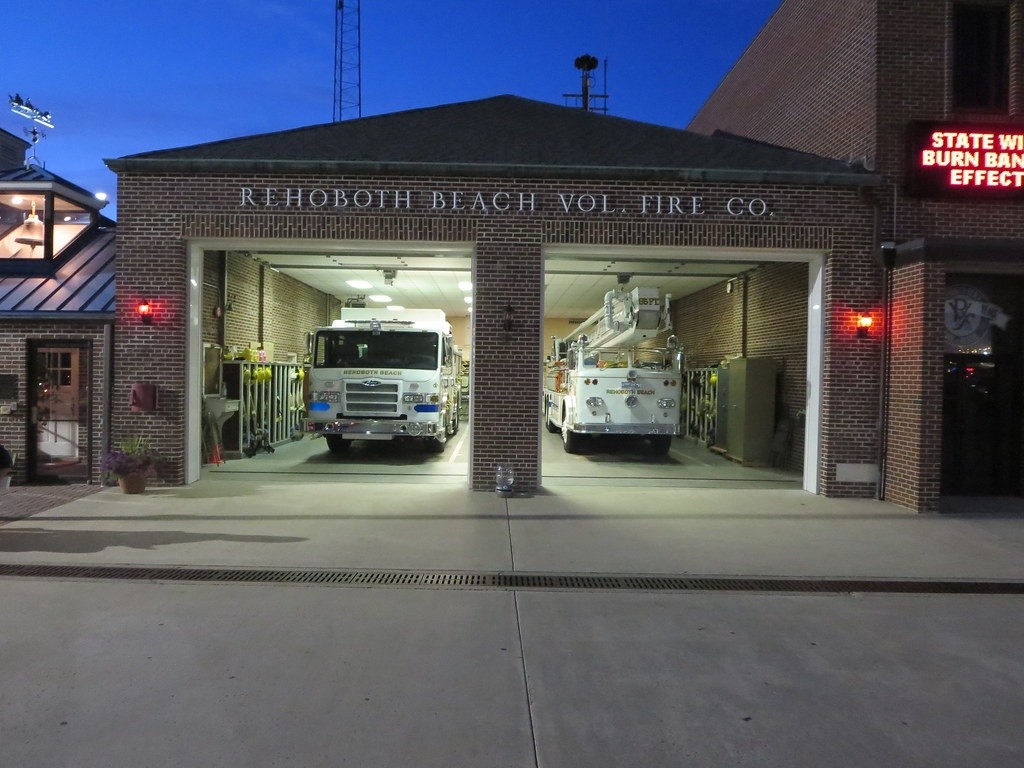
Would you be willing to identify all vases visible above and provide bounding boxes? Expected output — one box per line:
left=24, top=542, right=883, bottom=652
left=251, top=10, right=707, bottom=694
left=117, top=470, right=146, bottom=494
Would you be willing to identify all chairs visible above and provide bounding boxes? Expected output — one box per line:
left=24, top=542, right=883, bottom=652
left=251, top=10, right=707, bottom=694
left=327, top=343, right=357, bottom=364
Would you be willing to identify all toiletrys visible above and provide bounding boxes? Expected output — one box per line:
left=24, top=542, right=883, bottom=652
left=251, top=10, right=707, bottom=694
left=221, top=382, right=227, bottom=398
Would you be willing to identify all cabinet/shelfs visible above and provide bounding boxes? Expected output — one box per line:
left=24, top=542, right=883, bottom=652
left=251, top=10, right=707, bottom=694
left=223, top=360, right=307, bottom=458
left=709, top=358, right=777, bottom=466
left=682, top=365, right=716, bottom=447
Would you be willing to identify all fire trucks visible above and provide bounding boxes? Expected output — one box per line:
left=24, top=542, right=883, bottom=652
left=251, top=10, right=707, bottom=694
left=302, top=307, right=463, bottom=454
left=544, top=286, right=688, bottom=459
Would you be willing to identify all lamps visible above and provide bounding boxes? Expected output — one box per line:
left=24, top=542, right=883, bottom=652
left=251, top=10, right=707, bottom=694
left=382, top=269, right=397, bottom=286
left=617, top=275, right=629, bottom=285
left=138, top=297, right=152, bottom=324
left=855, top=311, right=872, bottom=337
left=504, top=300, right=515, bottom=330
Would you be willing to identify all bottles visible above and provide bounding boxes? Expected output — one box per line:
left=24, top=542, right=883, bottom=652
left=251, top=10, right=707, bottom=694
left=223, top=346, right=266, bottom=362
left=220, top=382, right=227, bottom=399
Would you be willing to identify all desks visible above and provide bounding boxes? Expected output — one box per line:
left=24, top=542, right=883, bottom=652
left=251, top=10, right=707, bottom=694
left=204, top=397, right=240, bottom=465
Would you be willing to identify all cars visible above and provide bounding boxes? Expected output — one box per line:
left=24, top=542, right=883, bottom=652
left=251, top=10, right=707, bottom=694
left=940, top=349, right=998, bottom=413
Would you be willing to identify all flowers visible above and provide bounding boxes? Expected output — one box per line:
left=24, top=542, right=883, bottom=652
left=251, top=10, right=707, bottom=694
left=102, top=430, right=154, bottom=480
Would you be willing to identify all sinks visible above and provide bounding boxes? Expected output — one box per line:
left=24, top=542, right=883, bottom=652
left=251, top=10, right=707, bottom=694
left=205, top=398, right=240, bottom=413
